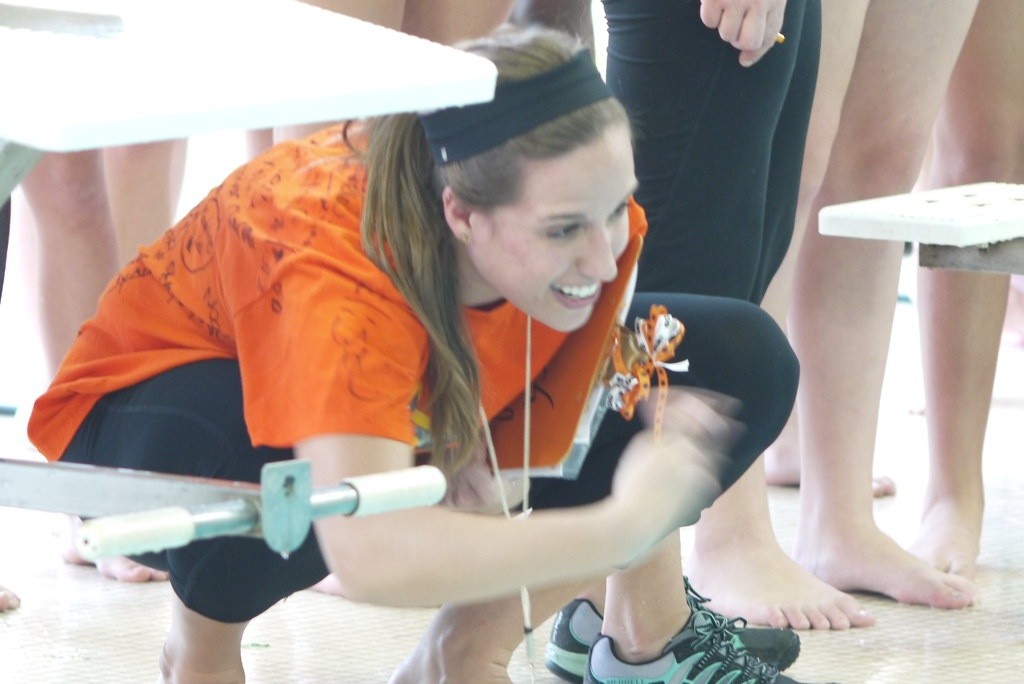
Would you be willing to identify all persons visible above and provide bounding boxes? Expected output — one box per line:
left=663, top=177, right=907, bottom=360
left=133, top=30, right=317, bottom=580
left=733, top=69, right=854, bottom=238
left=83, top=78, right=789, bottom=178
left=0, top=0, right=1024, bottom=684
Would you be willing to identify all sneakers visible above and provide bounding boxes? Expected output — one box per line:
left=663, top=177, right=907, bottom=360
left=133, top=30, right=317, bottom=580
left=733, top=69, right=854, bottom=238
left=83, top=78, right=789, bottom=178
left=587, top=601, right=804, bottom=684
left=544, top=573, right=801, bottom=684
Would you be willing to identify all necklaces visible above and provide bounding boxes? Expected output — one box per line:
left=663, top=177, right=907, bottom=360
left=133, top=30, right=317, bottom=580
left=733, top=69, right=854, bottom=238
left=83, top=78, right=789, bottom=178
left=455, top=310, right=539, bottom=683
